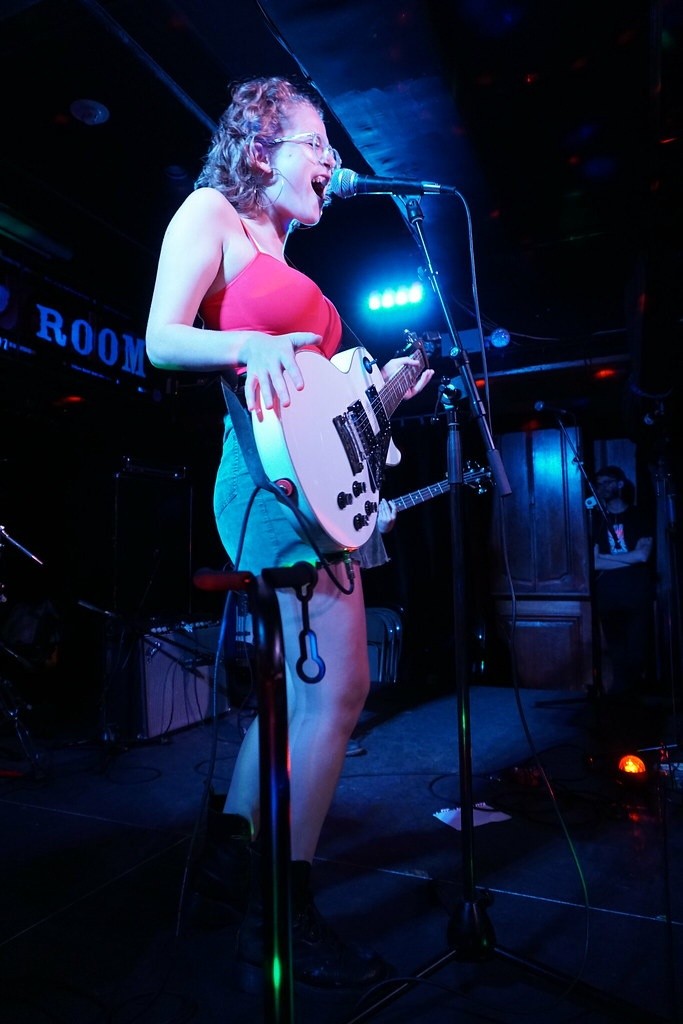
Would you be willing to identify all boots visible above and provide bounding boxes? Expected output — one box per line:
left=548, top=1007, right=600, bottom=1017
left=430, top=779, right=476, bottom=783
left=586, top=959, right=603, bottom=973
left=232, top=839, right=388, bottom=995
left=193, top=795, right=267, bottom=932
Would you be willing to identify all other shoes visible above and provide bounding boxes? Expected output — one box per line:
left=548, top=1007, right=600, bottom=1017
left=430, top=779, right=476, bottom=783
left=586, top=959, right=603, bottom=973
left=346, top=740, right=366, bottom=759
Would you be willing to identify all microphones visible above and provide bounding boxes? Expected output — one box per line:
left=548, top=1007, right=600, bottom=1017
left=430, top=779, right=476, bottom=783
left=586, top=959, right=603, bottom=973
left=184, top=655, right=224, bottom=667
left=331, top=168, right=456, bottom=199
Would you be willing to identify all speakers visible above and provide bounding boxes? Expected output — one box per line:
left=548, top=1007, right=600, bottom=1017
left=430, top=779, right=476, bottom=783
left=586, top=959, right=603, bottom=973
left=102, top=612, right=232, bottom=743
left=113, top=473, right=193, bottom=624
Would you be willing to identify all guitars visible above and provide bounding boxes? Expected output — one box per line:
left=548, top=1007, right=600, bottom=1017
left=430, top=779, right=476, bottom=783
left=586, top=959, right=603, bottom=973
left=250, top=327, right=429, bottom=560
left=377, top=458, right=496, bottom=520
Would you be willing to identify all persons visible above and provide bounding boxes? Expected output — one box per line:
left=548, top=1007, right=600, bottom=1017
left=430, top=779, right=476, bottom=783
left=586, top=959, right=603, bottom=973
left=144, top=76, right=436, bottom=1003
left=592, top=466, right=657, bottom=713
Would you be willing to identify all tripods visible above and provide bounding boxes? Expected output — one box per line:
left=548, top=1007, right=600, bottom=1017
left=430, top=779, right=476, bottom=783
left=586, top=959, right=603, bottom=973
left=324, top=192, right=677, bottom=1024
